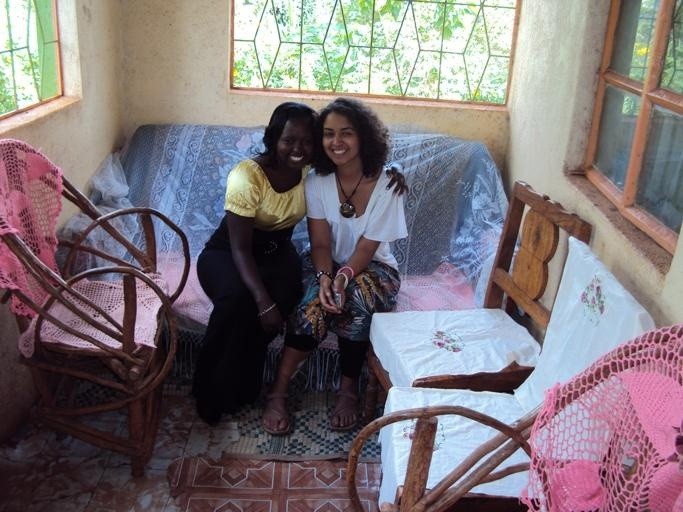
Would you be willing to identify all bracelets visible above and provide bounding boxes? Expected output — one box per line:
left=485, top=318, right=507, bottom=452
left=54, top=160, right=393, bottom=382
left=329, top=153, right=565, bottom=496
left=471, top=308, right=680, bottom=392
left=333, top=272, right=350, bottom=291
left=258, top=303, right=275, bottom=321
left=315, top=269, right=331, bottom=281
left=336, top=264, right=354, bottom=282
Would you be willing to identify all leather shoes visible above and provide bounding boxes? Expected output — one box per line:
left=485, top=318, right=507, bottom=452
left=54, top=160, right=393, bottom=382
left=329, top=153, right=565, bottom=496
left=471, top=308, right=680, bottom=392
left=191, top=383, right=221, bottom=426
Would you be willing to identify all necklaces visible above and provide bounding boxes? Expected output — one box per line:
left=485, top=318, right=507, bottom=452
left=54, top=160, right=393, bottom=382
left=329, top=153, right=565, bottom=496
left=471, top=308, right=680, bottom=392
left=331, top=167, right=368, bottom=218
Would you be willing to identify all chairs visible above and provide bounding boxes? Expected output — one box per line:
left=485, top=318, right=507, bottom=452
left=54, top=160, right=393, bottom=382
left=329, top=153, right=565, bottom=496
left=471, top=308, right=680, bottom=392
left=1, top=139, right=192, bottom=478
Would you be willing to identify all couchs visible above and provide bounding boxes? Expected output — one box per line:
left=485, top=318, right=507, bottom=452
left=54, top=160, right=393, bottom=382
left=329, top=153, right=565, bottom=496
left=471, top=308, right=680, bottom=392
left=57, top=123, right=520, bottom=393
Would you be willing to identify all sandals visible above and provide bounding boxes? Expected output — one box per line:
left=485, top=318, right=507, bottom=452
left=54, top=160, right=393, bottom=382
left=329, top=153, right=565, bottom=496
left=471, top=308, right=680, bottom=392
left=260, top=392, right=291, bottom=435
left=329, top=391, right=359, bottom=431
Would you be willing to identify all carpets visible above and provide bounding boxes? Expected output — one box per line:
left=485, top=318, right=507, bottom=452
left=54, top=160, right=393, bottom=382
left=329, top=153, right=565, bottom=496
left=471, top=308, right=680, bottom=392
left=205, top=380, right=383, bottom=462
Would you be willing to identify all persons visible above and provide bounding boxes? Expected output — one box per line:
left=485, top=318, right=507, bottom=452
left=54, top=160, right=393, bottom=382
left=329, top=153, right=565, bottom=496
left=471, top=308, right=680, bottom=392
left=190, top=100, right=409, bottom=427
left=258, top=98, right=409, bottom=437
